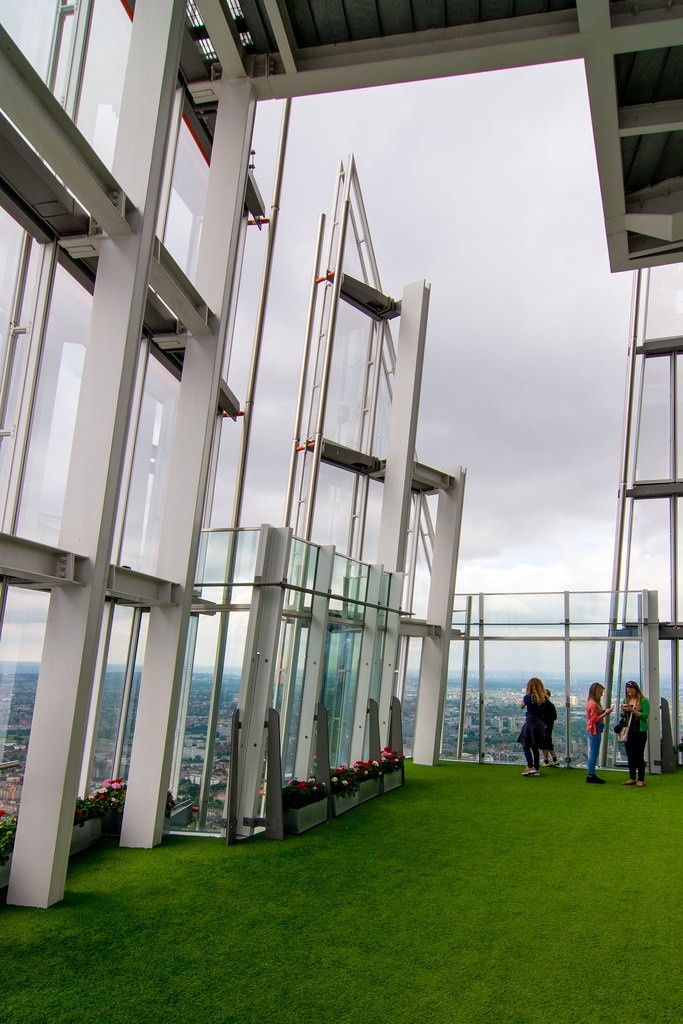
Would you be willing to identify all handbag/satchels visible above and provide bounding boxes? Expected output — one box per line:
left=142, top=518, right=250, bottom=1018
left=614, top=716, right=626, bottom=734
left=617, top=727, right=630, bottom=742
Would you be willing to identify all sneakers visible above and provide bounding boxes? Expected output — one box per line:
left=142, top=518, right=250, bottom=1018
left=540, top=763, right=550, bottom=766
left=522, top=766, right=537, bottom=775
left=534, top=770, right=540, bottom=776
left=551, top=761, right=559, bottom=766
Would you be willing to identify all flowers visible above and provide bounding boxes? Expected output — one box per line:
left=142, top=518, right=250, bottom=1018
left=329, top=746, right=405, bottom=799
left=0, top=778, right=175, bottom=866
left=282, top=776, right=328, bottom=809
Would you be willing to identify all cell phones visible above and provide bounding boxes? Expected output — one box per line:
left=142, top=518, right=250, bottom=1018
left=621, top=705, right=634, bottom=708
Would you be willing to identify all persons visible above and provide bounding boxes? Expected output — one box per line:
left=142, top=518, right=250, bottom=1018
left=586, top=683, right=614, bottom=784
left=620, top=681, right=650, bottom=787
left=516, top=678, right=559, bottom=776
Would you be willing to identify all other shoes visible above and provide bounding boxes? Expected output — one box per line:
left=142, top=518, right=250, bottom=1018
left=634, top=782, right=646, bottom=788
left=622, top=779, right=636, bottom=785
left=587, top=776, right=605, bottom=784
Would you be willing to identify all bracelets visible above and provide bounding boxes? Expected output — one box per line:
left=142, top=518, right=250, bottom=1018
left=603, top=711, right=608, bottom=717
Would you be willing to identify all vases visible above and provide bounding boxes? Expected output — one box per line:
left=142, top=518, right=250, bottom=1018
left=101, top=811, right=171, bottom=838
left=0, top=855, right=13, bottom=888
left=283, top=797, right=327, bottom=834
left=68, top=814, right=110, bottom=859
left=332, top=768, right=402, bottom=816
